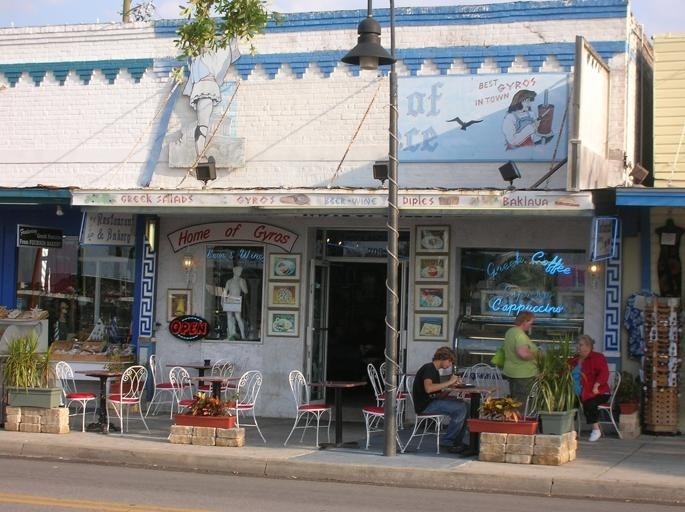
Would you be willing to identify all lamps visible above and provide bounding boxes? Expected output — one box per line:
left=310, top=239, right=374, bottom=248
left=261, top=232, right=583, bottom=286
left=56, top=204, right=64, bottom=216
left=499, top=160, right=521, bottom=187
left=196, top=162, right=217, bottom=189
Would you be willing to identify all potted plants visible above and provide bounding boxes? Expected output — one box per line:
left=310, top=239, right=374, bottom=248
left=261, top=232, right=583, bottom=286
left=617, top=371, right=637, bottom=414
left=2, top=334, right=62, bottom=409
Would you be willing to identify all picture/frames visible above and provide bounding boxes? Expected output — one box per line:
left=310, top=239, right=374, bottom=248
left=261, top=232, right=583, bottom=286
left=268, top=253, right=302, bottom=281
left=413, top=283, right=448, bottom=312
left=413, top=254, right=450, bottom=284
left=413, top=312, right=448, bottom=342
left=167, top=288, right=193, bottom=322
left=266, top=310, right=300, bottom=336
left=414, top=224, right=450, bottom=254
left=268, top=281, right=301, bottom=309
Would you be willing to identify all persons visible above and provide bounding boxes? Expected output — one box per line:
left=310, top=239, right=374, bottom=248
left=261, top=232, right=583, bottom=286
left=567, top=335, right=611, bottom=442
left=223, top=266, right=248, bottom=340
left=501, top=311, right=545, bottom=416
left=502, top=90, right=541, bottom=150
left=413, top=347, right=471, bottom=454
left=654, top=219, right=685, bottom=297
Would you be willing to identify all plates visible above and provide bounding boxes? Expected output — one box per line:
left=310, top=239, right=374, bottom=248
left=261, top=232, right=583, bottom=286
left=275, top=261, right=295, bottom=276
left=422, top=236, right=443, bottom=249
left=420, top=323, right=441, bottom=336
left=421, top=266, right=444, bottom=277
left=454, top=383, right=475, bottom=388
left=421, top=295, right=443, bottom=306
left=273, top=319, right=293, bottom=331
left=277, top=288, right=294, bottom=303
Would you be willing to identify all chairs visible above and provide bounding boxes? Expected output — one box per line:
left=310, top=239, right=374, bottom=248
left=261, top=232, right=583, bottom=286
left=54, top=354, right=266, bottom=443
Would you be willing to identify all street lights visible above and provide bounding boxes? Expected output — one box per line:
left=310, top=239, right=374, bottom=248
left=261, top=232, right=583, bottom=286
left=341, top=1, right=400, bottom=456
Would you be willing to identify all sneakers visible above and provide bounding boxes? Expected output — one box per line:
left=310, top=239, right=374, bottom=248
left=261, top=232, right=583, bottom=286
left=447, top=443, right=479, bottom=456
left=589, top=428, right=601, bottom=442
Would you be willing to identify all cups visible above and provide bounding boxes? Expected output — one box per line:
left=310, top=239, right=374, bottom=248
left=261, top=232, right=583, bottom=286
left=203, top=359, right=211, bottom=367
left=466, top=303, right=471, bottom=315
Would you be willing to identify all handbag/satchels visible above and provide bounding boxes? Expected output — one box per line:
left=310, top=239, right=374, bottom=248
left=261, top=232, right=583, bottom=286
left=490, top=347, right=505, bottom=367
